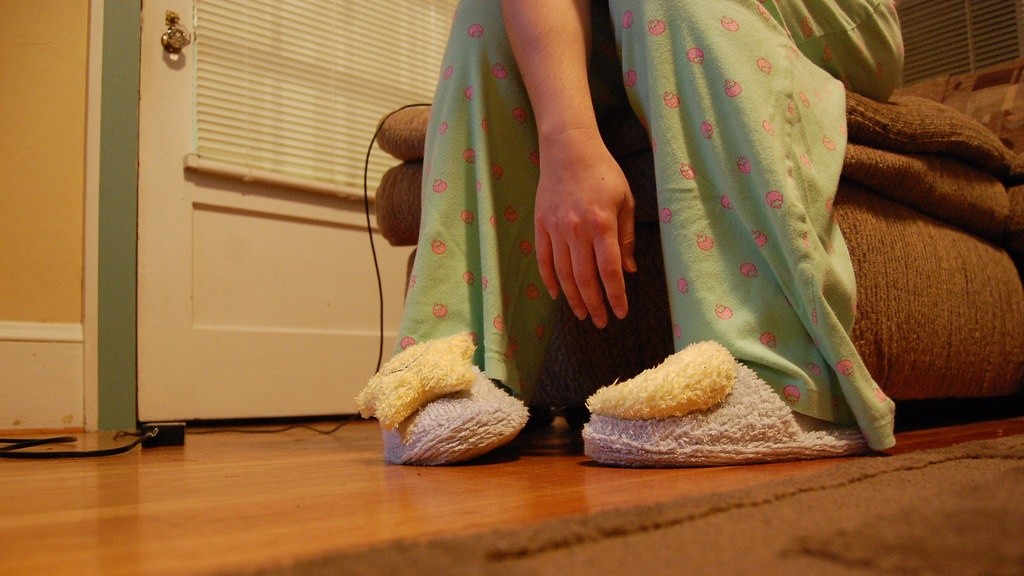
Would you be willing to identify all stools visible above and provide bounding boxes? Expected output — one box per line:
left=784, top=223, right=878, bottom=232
left=373, top=97, right=1024, bottom=412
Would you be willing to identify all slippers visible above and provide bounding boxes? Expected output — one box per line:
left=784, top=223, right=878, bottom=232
left=355, top=332, right=531, bottom=468
left=580, top=341, right=866, bottom=465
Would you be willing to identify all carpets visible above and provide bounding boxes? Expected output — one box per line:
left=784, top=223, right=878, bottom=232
left=248, top=432, right=1024, bottom=576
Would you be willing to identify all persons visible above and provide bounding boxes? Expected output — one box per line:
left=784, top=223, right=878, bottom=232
left=390, top=2, right=900, bottom=464
